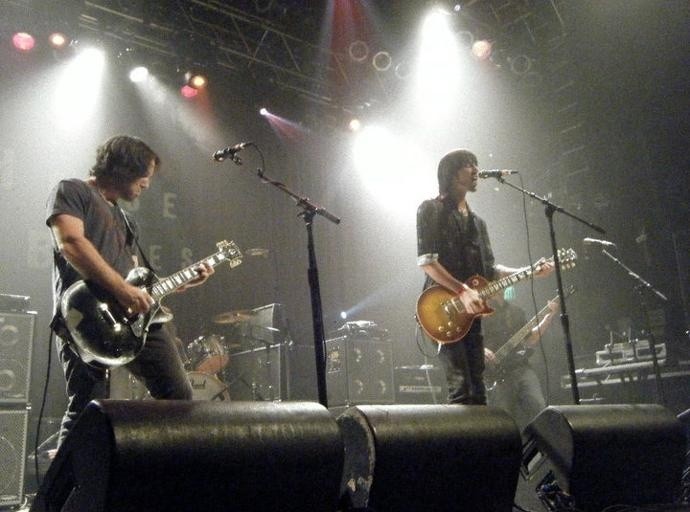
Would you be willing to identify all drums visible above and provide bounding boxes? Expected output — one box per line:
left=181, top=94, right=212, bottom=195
left=187, top=333, right=228, bottom=371
left=138, top=371, right=231, bottom=401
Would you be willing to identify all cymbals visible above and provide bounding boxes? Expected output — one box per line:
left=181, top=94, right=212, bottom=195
left=212, top=310, right=260, bottom=327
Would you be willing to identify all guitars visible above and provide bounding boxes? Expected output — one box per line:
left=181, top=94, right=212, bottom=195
left=414, top=247, right=576, bottom=345
left=477, top=285, right=576, bottom=390
left=53, top=239, right=243, bottom=366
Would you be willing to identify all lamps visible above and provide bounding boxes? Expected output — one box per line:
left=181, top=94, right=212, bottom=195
left=71, top=27, right=206, bottom=95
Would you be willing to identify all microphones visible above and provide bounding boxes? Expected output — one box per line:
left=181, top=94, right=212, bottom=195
left=478, top=169, right=519, bottom=178
left=583, top=237, right=614, bottom=249
left=212, top=143, right=247, bottom=162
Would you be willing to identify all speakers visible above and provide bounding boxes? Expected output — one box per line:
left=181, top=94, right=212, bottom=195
left=335, top=403, right=522, bottom=512
left=29, top=399, right=345, bottom=512
left=0, top=409, right=29, bottom=507
left=250, top=302, right=286, bottom=348
left=0, top=311, right=39, bottom=406
left=513, top=404, right=684, bottom=512
left=217, top=343, right=288, bottom=401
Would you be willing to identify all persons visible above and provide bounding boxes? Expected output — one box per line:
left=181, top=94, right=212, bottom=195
left=415, top=149, right=554, bottom=405
left=48, top=137, right=214, bottom=452
left=479, top=278, right=546, bottom=422
